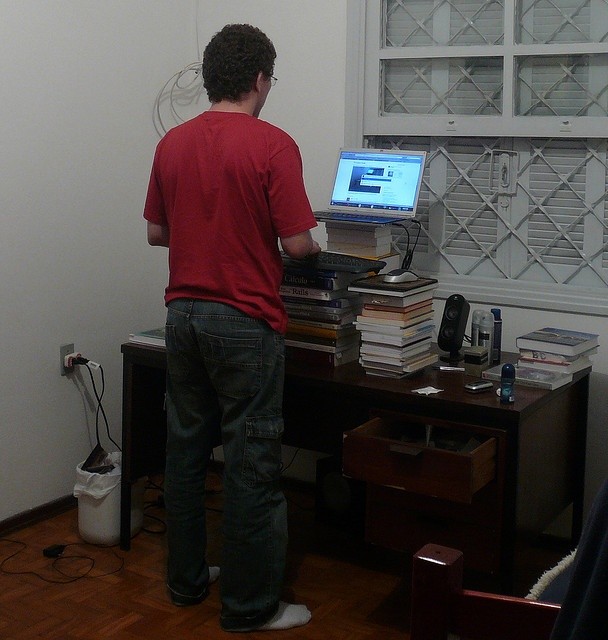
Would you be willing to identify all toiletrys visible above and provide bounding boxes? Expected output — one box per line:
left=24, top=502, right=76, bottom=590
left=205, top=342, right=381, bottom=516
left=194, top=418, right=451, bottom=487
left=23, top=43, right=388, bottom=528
left=500, top=363, right=516, bottom=404
left=478, top=313, right=495, bottom=365
left=471, top=310, right=484, bottom=347
left=490, top=308, right=503, bottom=363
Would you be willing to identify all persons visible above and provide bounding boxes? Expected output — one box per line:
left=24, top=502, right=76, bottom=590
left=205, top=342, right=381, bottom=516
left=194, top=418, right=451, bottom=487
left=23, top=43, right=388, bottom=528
left=142, top=24, right=321, bottom=631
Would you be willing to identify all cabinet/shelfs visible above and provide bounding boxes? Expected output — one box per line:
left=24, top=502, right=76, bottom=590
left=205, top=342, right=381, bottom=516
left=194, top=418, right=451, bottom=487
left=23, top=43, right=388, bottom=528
left=340, top=340, right=593, bottom=586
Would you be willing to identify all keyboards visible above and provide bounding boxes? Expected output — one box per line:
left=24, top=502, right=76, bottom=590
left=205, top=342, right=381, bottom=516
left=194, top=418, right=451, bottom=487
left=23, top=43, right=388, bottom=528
left=279, top=251, right=386, bottom=273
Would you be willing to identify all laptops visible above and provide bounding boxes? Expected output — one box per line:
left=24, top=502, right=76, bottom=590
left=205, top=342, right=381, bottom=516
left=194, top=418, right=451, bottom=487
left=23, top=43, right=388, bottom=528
left=312, top=148, right=426, bottom=223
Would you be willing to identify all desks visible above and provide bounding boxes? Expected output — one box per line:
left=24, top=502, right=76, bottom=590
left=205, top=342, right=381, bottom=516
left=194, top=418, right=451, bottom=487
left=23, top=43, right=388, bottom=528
left=120, top=341, right=351, bottom=550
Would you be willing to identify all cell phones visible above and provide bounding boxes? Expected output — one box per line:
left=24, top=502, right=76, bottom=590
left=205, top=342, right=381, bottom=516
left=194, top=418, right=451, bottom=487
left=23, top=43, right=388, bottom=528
left=464, top=379, right=495, bottom=394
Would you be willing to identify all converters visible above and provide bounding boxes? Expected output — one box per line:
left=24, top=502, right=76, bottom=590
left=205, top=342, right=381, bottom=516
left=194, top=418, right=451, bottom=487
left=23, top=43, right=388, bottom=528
left=43, top=545, right=64, bottom=558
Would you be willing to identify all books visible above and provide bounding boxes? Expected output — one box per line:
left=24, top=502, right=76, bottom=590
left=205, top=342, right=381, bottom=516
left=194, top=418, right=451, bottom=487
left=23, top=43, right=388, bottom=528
left=519, top=345, right=600, bottom=365
left=517, top=357, right=593, bottom=374
left=128, top=328, right=168, bottom=347
left=348, top=274, right=440, bottom=381
left=277, top=265, right=362, bottom=370
left=324, top=220, right=401, bottom=277
left=516, top=327, right=600, bottom=357
left=482, top=361, right=573, bottom=391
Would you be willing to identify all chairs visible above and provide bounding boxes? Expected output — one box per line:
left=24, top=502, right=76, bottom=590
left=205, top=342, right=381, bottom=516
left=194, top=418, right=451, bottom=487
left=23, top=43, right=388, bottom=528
left=413, top=481, right=607, bottom=638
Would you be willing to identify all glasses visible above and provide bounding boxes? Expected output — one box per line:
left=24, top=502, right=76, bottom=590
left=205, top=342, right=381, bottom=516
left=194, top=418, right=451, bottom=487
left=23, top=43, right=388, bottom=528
left=268, top=75, right=277, bottom=86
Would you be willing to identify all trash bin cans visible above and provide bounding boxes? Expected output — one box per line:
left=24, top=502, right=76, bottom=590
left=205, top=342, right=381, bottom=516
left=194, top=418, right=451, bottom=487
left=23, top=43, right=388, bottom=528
left=75, top=452, right=143, bottom=546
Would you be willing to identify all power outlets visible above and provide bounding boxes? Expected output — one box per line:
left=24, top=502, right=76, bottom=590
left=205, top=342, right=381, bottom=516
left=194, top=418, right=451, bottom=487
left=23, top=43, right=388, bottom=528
left=60, top=343, right=75, bottom=376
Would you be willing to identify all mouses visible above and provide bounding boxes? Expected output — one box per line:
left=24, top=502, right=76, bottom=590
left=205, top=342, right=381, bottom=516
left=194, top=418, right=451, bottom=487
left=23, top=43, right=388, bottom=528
left=383, top=268, right=418, bottom=283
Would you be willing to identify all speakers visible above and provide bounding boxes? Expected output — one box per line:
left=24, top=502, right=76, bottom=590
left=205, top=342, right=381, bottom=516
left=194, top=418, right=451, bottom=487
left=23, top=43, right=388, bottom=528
left=438, top=293, right=470, bottom=362
